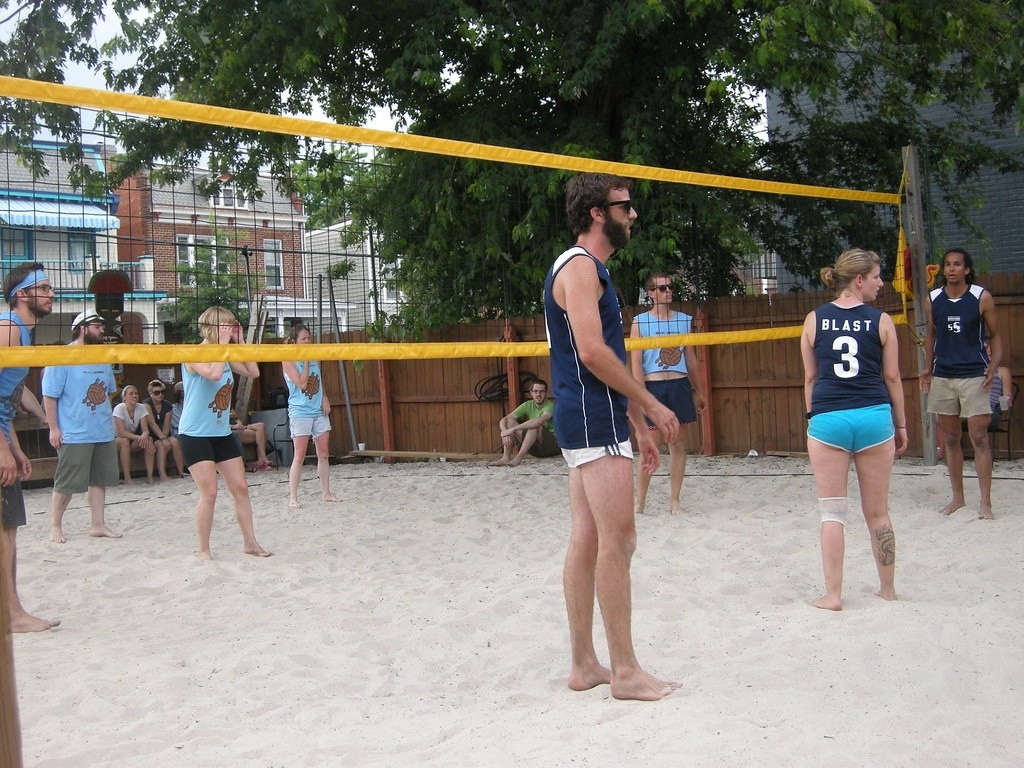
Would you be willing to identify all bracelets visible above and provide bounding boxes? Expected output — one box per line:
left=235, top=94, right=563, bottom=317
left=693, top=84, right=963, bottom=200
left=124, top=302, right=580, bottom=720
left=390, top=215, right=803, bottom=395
left=895, top=425, right=907, bottom=428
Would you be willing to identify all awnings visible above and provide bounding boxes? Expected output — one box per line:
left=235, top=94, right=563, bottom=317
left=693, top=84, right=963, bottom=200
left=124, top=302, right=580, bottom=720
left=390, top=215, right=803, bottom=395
left=0, top=197, right=120, bottom=232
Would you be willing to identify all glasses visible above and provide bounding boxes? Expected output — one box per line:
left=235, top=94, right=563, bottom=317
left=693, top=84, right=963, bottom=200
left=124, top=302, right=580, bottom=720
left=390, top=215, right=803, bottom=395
left=530, top=388, right=547, bottom=394
left=605, top=199, right=631, bottom=214
left=85, top=324, right=105, bottom=330
left=152, top=390, right=164, bottom=396
left=21, top=283, right=53, bottom=293
left=652, top=283, right=675, bottom=292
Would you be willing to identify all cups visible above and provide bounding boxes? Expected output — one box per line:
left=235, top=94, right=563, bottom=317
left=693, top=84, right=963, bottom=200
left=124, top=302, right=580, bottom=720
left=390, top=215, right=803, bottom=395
left=439, top=458, right=446, bottom=462
left=998, top=396, right=1010, bottom=411
left=358, top=443, right=366, bottom=450
left=747, top=449, right=758, bottom=458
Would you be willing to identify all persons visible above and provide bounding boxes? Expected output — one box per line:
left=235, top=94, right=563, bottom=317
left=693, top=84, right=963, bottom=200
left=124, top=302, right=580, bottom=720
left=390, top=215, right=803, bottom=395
left=177, top=306, right=275, bottom=561
left=280, top=325, right=342, bottom=508
left=113, top=379, right=274, bottom=486
left=917, top=248, right=1002, bottom=522
left=542, top=172, right=682, bottom=702
left=488, top=379, right=563, bottom=466
left=629, top=274, right=708, bottom=516
left=0, top=262, right=62, bottom=633
left=41, top=310, right=123, bottom=543
left=801, top=248, right=910, bottom=612
left=983, top=336, right=1013, bottom=428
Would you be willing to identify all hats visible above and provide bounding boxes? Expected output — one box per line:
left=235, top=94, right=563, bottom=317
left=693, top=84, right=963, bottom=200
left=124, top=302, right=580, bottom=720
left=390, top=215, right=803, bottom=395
left=70, top=307, right=106, bottom=332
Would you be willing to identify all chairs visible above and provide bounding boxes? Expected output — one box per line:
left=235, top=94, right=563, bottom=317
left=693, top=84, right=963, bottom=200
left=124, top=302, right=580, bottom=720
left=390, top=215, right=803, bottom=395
left=272, top=416, right=318, bottom=471
left=961, top=382, right=1018, bottom=468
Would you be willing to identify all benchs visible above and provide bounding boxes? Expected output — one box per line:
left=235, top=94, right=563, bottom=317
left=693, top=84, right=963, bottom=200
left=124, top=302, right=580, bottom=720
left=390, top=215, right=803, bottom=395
left=13, top=415, right=187, bottom=481
